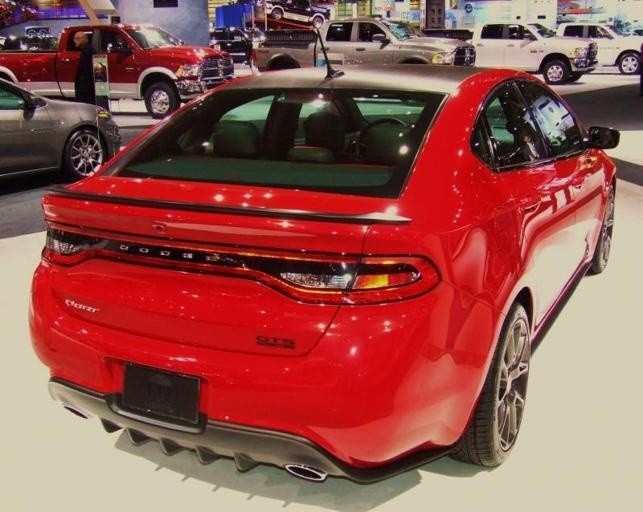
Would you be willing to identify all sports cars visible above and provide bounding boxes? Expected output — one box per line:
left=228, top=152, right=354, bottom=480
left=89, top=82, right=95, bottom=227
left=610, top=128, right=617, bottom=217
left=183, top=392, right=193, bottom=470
left=23, top=26, right=49, bottom=35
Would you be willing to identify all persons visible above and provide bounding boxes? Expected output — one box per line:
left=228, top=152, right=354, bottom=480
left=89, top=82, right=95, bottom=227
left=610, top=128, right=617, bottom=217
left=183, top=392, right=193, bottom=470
left=74, top=30, right=96, bottom=103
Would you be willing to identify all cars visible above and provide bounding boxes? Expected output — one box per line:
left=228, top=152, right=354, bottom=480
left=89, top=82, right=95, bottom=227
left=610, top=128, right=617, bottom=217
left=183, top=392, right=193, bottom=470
left=0, top=78, right=122, bottom=195
left=207, top=27, right=250, bottom=65
left=256, top=0, right=331, bottom=29
left=25, top=26, right=622, bottom=487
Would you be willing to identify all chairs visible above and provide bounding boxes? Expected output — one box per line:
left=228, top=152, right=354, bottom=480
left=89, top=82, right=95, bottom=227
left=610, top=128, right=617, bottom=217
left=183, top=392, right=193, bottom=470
left=302, top=107, right=348, bottom=162
left=208, top=117, right=264, bottom=156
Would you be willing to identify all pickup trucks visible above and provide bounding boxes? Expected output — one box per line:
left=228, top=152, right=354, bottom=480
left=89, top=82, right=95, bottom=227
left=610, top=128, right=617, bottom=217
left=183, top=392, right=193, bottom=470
left=422, top=22, right=599, bottom=85
left=249, top=18, right=477, bottom=73
left=0, top=24, right=235, bottom=120
left=550, top=22, right=643, bottom=74
